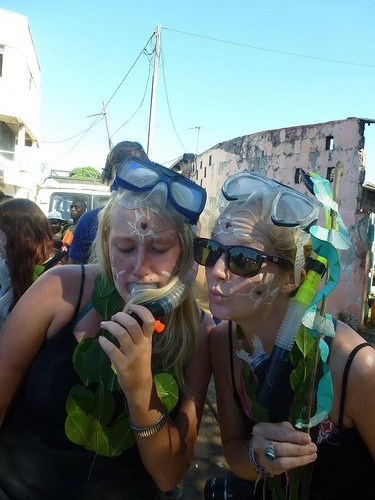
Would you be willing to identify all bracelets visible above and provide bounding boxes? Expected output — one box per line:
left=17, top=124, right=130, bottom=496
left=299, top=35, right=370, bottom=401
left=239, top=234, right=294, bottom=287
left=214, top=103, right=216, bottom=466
left=130, top=406, right=168, bottom=438
left=246, top=439, right=269, bottom=476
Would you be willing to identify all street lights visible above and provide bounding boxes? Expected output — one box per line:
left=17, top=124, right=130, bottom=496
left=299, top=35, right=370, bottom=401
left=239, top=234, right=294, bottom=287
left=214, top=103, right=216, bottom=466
left=186, top=125, right=202, bottom=154
left=86, top=100, right=112, bottom=151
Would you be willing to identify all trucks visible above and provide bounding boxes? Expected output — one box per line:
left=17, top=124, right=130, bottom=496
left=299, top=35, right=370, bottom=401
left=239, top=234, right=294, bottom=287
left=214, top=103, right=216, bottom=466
left=34, top=168, right=113, bottom=224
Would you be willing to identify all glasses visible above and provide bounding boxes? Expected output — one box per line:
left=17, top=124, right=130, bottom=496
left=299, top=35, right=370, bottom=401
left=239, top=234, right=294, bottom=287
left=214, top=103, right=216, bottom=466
left=193, top=238, right=292, bottom=278
left=110, top=156, right=208, bottom=225
left=70, top=204, right=81, bottom=209
left=221, top=170, right=320, bottom=235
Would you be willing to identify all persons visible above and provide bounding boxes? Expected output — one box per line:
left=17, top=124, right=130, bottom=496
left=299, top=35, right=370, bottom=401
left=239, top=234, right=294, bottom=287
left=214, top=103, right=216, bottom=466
left=205, top=170, right=375, bottom=500
left=0, top=141, right=216, bottom=500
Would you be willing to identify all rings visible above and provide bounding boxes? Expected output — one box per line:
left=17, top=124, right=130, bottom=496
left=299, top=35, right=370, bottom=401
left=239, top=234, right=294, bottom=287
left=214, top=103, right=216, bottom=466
left=264, top=441, right=278, bottom=461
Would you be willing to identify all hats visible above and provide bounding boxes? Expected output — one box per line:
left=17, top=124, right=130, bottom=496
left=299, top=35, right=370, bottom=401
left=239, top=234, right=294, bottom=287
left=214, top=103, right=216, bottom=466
left=46, top=211, right=65, bottom=221
